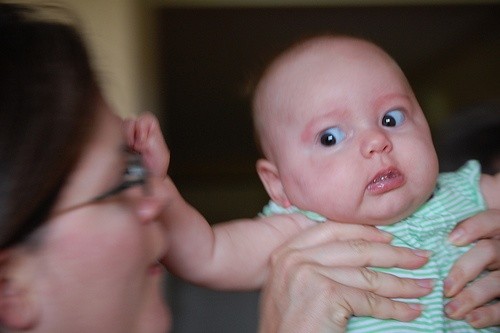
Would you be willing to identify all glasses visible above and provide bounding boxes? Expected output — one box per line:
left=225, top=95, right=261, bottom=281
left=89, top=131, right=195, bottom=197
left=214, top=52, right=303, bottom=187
left=43, top=146, right=147, bottom=222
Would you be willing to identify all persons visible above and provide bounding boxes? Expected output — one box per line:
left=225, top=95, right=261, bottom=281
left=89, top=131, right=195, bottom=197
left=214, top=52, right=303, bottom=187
left=2, top=18, right=499, bottom=333
left=125, top=37, right=500, bottom=332
